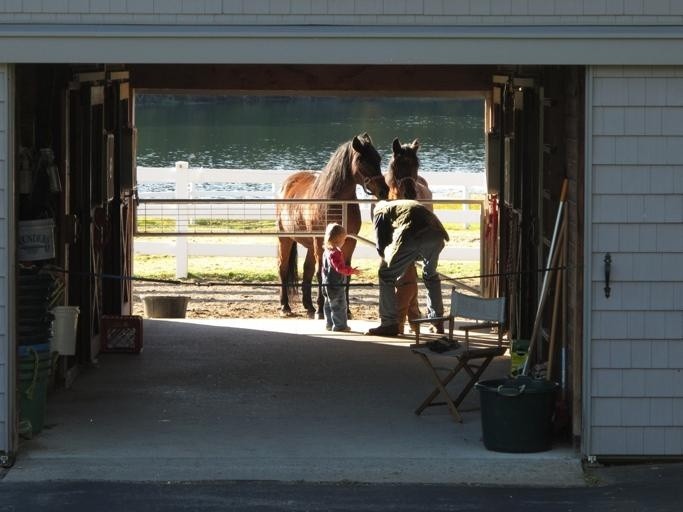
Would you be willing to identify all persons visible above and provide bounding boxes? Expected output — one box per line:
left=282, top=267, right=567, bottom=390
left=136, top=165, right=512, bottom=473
left=369, top=199, right=449, bottom=336
left=322, top=224, right=365, bottom=331
left=394, top=263, right=422, bottom=334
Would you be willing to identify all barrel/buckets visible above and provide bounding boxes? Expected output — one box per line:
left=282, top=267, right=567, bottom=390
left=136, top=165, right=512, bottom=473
left=16, top=273, right=55, bottom=430
left=51, top=305, right=81, bottom=355
left=472, top=376, right=558, bottom=453
left=17, top=218, right=57, bottom=261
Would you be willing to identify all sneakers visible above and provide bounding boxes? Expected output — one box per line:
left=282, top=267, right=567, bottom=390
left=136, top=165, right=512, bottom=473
left=327, top=323, right=352, bottom=333
left=429, top=324, right=444, bottom=334
left=368, top=325, right=397, bottom=335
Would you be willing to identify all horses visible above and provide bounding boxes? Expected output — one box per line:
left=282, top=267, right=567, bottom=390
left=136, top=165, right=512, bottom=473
left=371, top=136, right=432, bottom=217
left=274, top=130, right=390, bottom=317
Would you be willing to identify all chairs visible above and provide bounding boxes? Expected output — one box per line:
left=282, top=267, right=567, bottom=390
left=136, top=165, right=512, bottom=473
left=409, top=285, right=506, bottom=423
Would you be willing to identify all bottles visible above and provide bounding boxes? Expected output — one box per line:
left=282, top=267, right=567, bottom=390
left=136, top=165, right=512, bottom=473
left=37, top=148, right=63, bottom=196
left=16, top=149, right=35, bottom=193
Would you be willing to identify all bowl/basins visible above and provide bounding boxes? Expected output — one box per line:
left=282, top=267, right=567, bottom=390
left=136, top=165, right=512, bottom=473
left=140, top=295, right=191, bottom=318
